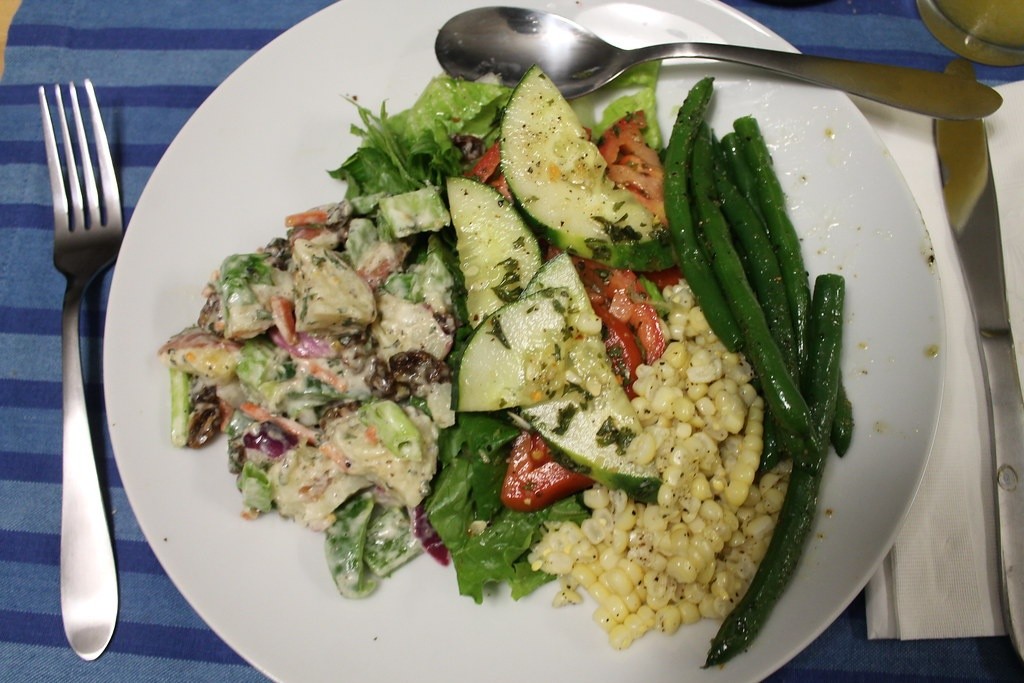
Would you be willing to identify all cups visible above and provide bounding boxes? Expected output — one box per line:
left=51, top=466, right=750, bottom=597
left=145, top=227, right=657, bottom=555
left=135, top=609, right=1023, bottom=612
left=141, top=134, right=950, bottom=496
left=915, top=0, right=1023, bottom=67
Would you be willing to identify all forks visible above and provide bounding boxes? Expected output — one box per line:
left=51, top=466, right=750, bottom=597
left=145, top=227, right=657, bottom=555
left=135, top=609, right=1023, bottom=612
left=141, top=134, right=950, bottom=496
left=39, top=79, right=124, bottom=663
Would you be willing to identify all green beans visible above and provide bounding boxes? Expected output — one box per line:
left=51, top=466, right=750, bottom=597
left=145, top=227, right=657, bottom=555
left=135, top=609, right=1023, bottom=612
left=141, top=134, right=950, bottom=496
left=660, top=73, right=853, bottom=665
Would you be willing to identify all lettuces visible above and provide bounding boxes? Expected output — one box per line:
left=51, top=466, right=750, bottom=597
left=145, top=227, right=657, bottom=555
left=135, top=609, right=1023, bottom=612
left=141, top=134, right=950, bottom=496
left=328, top=70, right=581, bottom=600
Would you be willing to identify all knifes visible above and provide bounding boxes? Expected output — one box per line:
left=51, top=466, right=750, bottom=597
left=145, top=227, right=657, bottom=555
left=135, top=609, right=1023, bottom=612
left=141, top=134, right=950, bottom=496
left=932, top=61, right=1023, bottom=662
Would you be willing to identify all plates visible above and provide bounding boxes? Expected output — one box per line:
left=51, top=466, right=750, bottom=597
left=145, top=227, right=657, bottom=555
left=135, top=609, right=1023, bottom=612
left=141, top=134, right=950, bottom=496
left=105, top=1, right=942, bottom=682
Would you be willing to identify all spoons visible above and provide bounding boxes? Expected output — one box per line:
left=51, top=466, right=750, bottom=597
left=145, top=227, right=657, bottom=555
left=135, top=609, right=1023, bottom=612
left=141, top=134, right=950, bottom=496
left=434, top=5, right=1003, bottom=120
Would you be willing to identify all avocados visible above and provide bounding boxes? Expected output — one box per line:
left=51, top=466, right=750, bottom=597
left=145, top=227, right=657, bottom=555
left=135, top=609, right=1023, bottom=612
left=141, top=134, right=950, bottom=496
left=447, top=63, right=680, bottom=508
